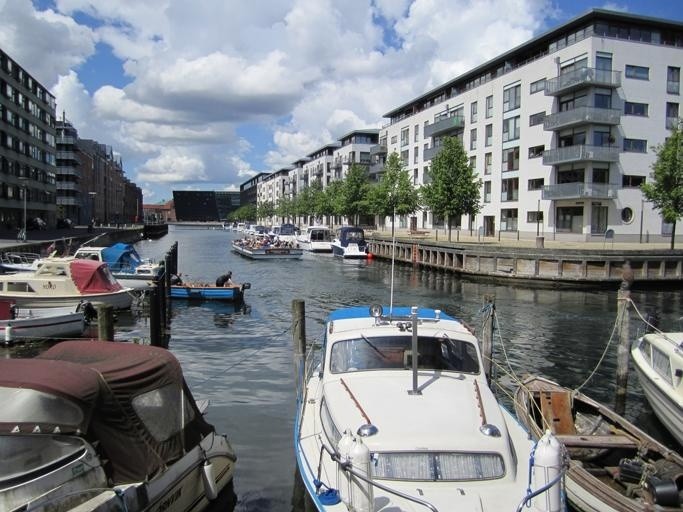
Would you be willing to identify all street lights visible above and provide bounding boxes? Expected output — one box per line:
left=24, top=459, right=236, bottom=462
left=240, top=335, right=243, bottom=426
left=17, top=175, right=32, bottom=244
left=86, top=190, right=100, bottom=233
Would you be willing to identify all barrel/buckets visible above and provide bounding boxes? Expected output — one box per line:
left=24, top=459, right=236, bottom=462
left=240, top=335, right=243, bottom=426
left=652, top=477, right=679, bottom=507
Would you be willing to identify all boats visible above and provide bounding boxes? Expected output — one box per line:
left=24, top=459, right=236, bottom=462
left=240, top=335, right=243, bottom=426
left=170, top=270, right=252, bottom=302
left=631, top=329, right=683, bottom=452
left=230, top=217, right=374, bottom=263
left=0, top=336, right=238, bottom=512
left=271, top=283, right=569, bottom=510
left=489, top=359, right=682, bottom=511
left=0, top=226, right=161, bottom=345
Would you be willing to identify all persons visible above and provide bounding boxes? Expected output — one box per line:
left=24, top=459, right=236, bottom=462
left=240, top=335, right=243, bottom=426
left=232, top=236, right=299, bottom=251
left=215, top=270, right=236, bottom=288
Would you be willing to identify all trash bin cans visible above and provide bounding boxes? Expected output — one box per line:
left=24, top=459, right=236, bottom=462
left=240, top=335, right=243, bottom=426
left=536, top=237, right=544, bottom=249
